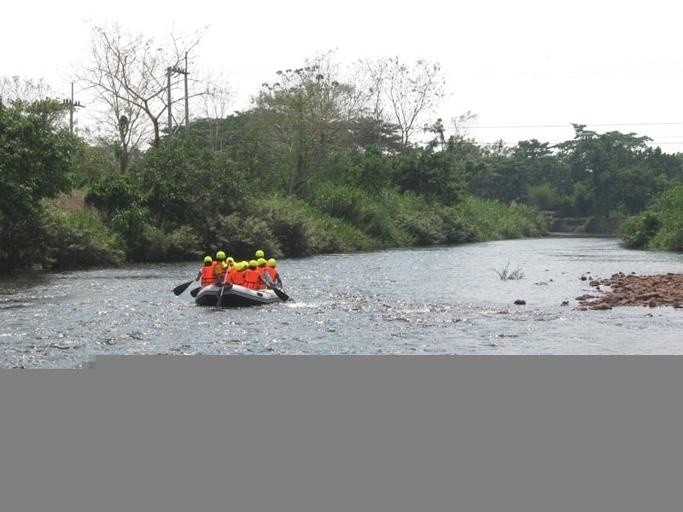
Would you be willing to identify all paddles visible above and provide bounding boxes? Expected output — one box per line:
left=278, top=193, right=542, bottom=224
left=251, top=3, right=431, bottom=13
left=272, top=286, right=289, bottom=301
left=172, top=278, right=196, bottom=297
left=190, top=287, right=202, bottom=298
left=217, top=264, right=231, bottom=307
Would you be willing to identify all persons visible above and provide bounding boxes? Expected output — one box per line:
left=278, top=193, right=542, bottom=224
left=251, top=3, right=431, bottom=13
left=196, top=250, right=283, bottom=290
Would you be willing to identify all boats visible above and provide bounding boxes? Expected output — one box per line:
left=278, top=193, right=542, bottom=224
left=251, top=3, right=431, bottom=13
left=194, top=279, right=287, bottom=307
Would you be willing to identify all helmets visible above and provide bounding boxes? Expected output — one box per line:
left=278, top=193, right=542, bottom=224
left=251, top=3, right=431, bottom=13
left=203, top=249, right=277, bottom=272
